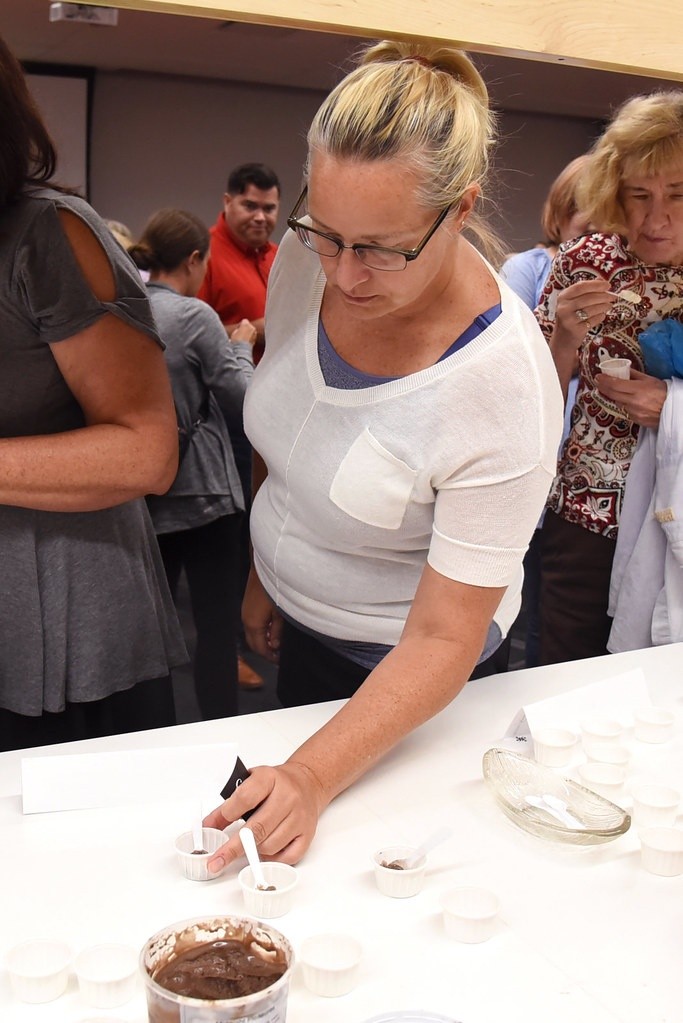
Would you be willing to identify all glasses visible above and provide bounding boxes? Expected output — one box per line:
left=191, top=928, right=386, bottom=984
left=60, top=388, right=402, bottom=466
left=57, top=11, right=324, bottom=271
left=287, top=184, right=460, bottom=271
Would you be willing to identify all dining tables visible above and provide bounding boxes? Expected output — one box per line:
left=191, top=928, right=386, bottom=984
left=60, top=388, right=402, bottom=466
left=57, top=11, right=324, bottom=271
left=0, top=643, right=682, bottom=1023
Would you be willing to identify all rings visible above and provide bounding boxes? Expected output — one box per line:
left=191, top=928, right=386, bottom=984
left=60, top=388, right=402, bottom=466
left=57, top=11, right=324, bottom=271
left=584, top=320, right=591, bottom=329
left=620, top=405, right=629, bottom=418
left=575, top=309, right=588, bottom=320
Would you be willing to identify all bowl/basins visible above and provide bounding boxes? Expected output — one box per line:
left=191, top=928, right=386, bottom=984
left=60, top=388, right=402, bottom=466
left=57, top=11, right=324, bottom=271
left=483, top=749, right=630, bottom=846
left=174, top=827, right=229, bottom=882
left=372, top=845, right=427, bottom=899
left=531, top=703, right=677, bottom=767
left=138, top=914, right=296, bottom=1023
left=237, top=862, right=301, bottom=920
left=598, top=359, right=632, bottom=380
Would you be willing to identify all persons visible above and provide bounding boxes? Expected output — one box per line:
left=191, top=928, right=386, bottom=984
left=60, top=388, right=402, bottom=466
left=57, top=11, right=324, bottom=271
left=0, top=37, right=179, bottom=752
left=194, top=162, right=281, bottom=689
left=201, top=38, right=565, bottom=874
left=125, top=207, right=258, bottom=722
left=498, top=155, right=597, bottom=669
left=532, top=88, right=683, bottom=667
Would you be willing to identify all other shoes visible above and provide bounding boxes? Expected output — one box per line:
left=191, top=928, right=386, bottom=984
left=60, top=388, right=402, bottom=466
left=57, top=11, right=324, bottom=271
left=235, top=657, right=263, bottom=689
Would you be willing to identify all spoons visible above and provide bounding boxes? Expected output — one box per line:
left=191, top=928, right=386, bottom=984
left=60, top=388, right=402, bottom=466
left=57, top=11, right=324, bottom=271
left=525, top=794, right=584, bottom=829
